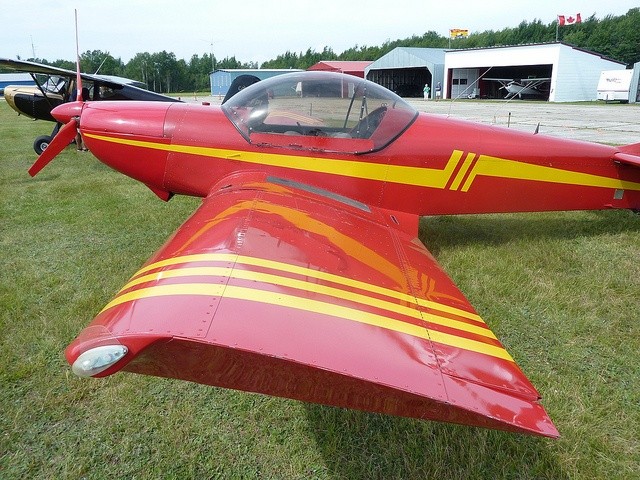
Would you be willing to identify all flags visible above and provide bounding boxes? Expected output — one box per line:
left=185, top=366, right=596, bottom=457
left=449, top=28, right=470, bottom=41
left=557, top=12, right=584, bottom=26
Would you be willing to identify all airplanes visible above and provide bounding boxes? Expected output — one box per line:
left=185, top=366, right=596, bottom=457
left=0, top=58, right=186, bottom=155
left=481, top=77, right=551, bottom=100
left=28, top=8, right=640, bottom=441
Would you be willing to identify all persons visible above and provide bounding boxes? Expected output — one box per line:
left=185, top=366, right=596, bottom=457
left=422, top=83, right=431, bottom=100
left=434, top=81, right=443, bottom=102
